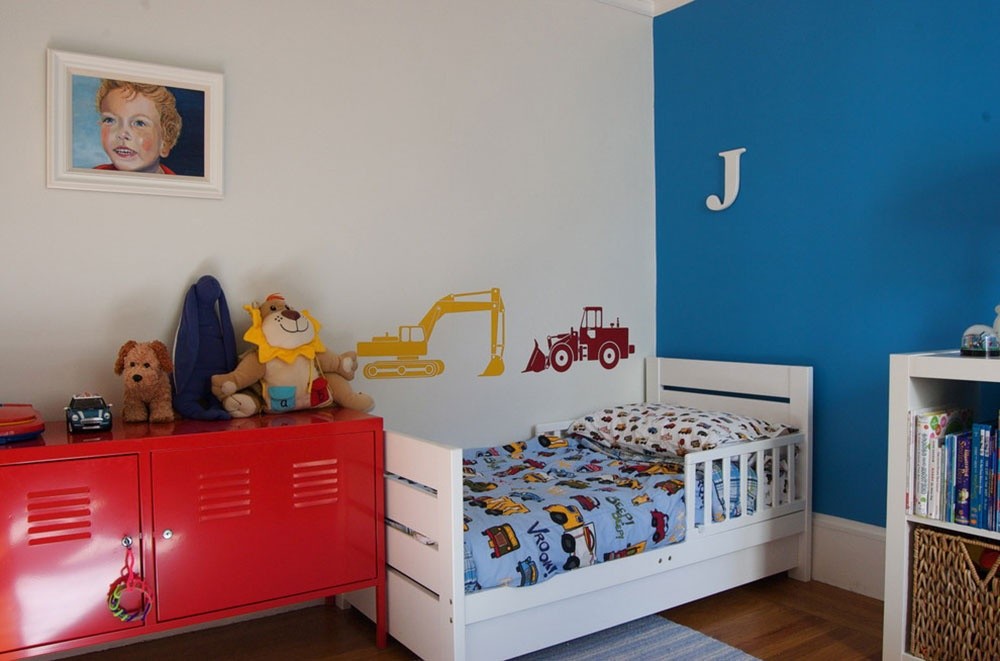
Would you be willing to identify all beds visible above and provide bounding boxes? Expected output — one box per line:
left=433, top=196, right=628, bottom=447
left=335, top=358, right=813, bottom=661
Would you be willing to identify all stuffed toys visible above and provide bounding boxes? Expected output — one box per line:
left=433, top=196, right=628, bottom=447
left=211, top=292, right=375, bottom=418
left=114, top=340, right=174, bottom=423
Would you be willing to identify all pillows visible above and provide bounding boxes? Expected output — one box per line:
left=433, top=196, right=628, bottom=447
left=568, top=403, right=799, bottom=461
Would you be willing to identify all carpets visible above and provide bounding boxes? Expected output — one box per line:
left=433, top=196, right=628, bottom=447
left=505, top=614, right=765, bottom=661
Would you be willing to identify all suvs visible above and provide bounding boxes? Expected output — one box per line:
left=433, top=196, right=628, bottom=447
left=63, top=392, right=113, bottom=433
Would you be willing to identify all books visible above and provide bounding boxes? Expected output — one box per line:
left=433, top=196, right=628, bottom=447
left=905, top=401, right=1000, bottom=533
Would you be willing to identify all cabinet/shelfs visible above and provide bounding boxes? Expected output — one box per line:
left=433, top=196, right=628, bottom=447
left=882, top=350, right=1000, bottom=661
left=0, top=415, right=146, bottom=661
left=145, top=405, right=387, bottom=648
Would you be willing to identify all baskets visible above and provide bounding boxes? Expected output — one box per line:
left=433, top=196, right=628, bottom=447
left=909, top=528, right=1000, bottom=661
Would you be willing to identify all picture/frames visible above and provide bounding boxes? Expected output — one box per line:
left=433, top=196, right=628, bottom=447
left=47, top=48, right=224, bottom=199
